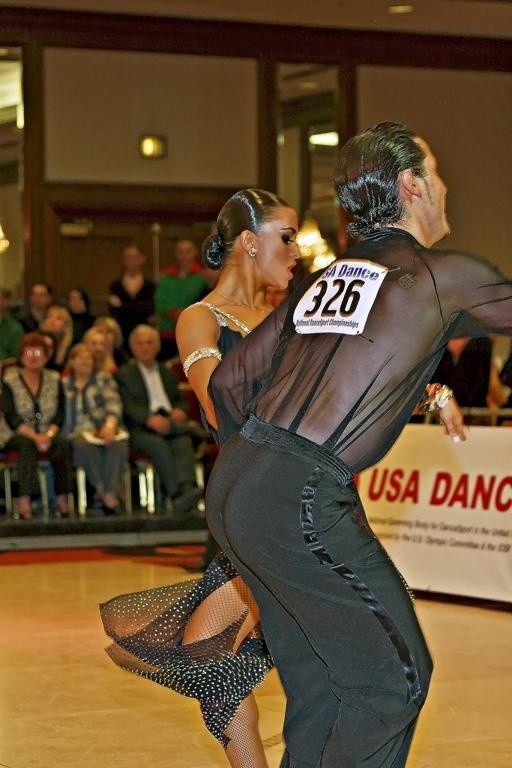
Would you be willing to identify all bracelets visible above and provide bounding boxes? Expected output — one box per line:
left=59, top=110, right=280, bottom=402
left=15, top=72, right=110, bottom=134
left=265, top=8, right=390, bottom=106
left=419, top=385, right=454, bottom=413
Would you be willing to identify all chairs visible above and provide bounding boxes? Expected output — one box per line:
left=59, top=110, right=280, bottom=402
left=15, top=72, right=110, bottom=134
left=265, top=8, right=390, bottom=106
left=1, top=353, right=215, bottom=522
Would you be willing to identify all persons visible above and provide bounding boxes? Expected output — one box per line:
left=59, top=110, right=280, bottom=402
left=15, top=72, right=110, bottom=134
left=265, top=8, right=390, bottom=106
left=0, top=237, right=217, bottom=521
left=98, top=189, right=465, bottom=767
left=267, top=286, right=291, bottom=310
left=205, top=120, right=512, bottom=768
left=409, top=335, right=511, bottom=423
